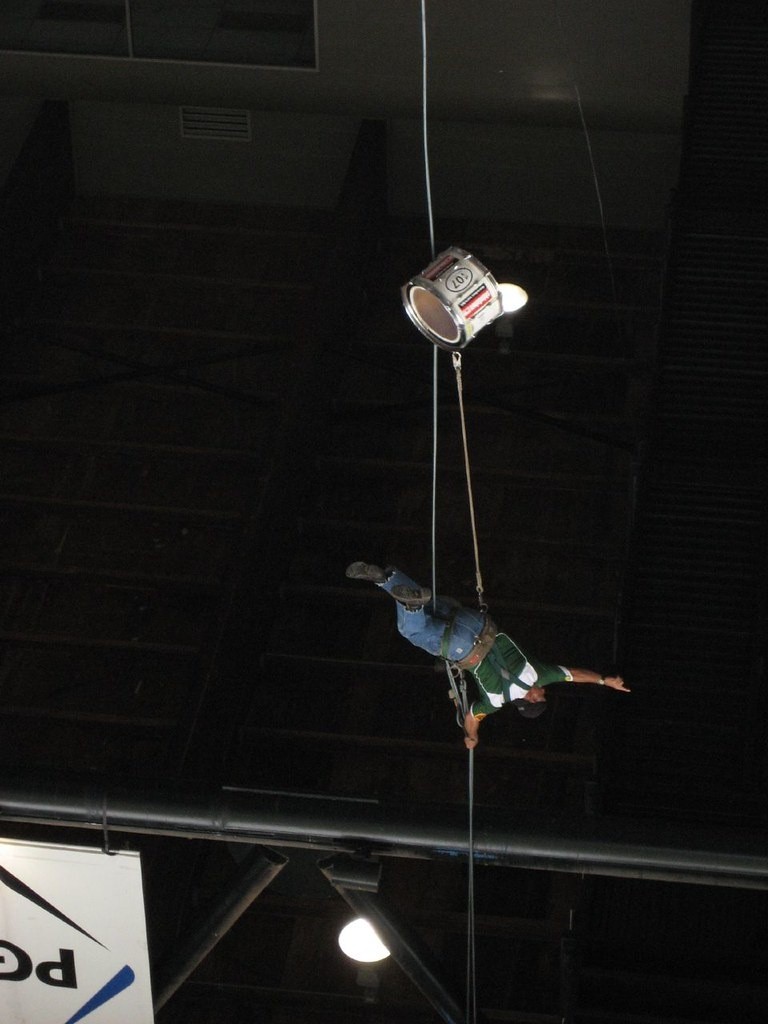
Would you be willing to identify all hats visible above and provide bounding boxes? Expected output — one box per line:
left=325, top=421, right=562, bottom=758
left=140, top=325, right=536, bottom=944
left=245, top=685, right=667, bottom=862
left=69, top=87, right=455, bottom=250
left=512, top=699, right=547, bottom=719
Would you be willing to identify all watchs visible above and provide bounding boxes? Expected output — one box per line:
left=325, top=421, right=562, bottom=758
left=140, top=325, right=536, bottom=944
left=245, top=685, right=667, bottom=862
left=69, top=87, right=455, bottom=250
left=597, top=673, right=606, bottom=686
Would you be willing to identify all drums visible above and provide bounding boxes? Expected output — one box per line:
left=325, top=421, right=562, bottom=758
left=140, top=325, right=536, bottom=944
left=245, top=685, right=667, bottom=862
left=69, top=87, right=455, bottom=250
left=401, top=245, right=506, bottom=352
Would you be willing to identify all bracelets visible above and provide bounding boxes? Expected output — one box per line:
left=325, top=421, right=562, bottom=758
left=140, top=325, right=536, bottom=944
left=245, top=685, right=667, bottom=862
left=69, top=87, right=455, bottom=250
left=468, top=737, right=478, bottom=743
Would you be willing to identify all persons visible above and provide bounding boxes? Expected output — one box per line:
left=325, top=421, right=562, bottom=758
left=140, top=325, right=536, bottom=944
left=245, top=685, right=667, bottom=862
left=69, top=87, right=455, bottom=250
left=344, top=560, right=631, bottom=749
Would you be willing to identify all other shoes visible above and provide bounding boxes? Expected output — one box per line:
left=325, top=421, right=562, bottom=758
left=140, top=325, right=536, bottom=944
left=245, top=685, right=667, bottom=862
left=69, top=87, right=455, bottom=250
left=346, top=562, right=385, bottom=582
left=391, top=584, right=431, bottom=604
